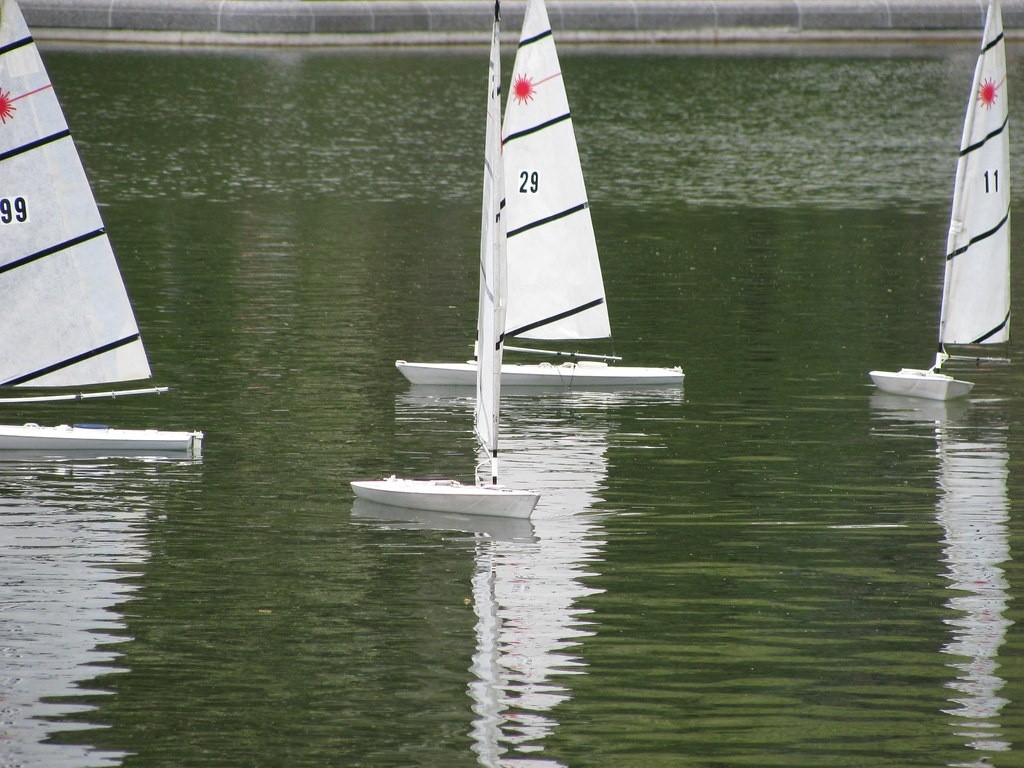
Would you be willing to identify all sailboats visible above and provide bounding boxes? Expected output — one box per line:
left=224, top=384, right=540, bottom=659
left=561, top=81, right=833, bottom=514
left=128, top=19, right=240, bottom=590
left=394, top=1, right=686, bottom=387
left=867, top=0, right=1013, bottom=401
left=1, top=2, right=206, bottom=458
left=350, top=1, right=543, bottom=522
